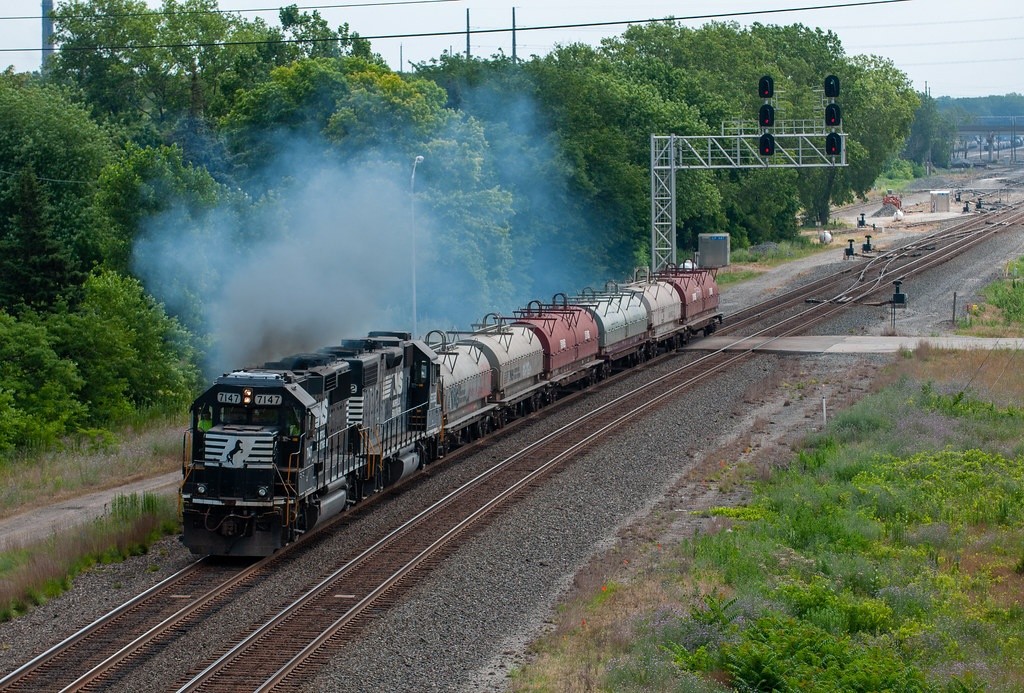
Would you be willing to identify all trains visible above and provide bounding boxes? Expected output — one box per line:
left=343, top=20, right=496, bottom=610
left=177, top=257, right=724, bottom=558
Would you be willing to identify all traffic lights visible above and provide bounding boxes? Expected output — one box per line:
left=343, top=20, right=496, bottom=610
left=826, top=133, right=841, bottom=156
left=824, top=74, right=840, bottom=98
left=825, top=103, right=841, bottom=127
left=758, top=104, right=774, bottom=128
left=758, top=75, right=774, bottom=99
left=758, top=133, right=774, bottom=156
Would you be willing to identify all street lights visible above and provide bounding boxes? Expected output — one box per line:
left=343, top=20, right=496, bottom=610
left=409, top=155, right=424, bottom=343
